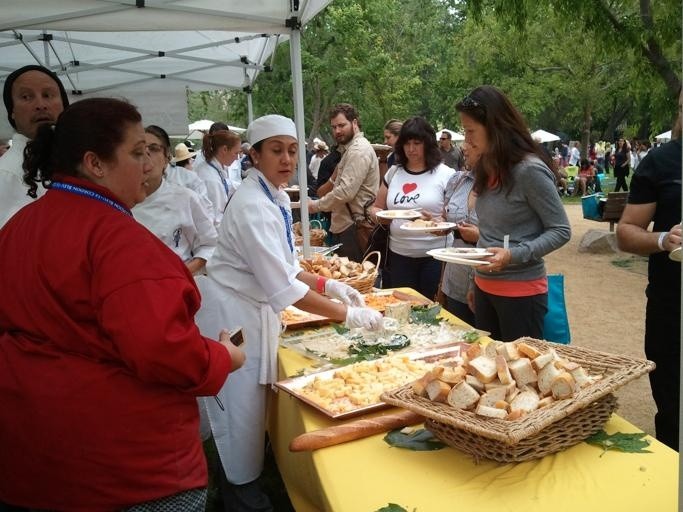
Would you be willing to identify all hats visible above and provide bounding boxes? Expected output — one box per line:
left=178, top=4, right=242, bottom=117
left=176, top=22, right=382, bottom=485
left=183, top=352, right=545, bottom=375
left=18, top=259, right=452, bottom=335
left=245, top=114, right=298, bottom=151
left=4, top=65, right=69, bottom=128
left=169, top=143, right=197, bottom=164
left=314, top=141, right=329, bottom=150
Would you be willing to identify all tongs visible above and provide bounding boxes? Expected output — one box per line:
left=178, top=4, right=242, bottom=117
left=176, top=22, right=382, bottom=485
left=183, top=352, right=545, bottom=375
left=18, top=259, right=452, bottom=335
left=317, top=243, right=343, bottom=256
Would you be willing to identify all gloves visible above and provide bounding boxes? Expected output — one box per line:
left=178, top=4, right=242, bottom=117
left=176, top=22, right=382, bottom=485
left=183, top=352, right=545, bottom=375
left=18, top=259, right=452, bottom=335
left=324, top=278, right=366, bottom=308
left=343, top=305, right=384, bottom=331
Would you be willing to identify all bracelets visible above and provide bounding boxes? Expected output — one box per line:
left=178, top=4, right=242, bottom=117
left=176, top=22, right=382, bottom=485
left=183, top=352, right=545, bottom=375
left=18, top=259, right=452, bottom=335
left=658, top=232, right=670, bottom=252
left=316, top=277, right=330, bottom=294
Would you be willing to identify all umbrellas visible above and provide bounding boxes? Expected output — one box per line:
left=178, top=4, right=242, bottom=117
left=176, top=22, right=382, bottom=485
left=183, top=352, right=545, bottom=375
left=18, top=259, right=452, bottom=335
left=529, top=128, right=561, bottom=145
left=168, top=117, right=248, bottom=141
left=434, top=127, right=466, bottom=142
left=654, top=129, right=673, bottom=144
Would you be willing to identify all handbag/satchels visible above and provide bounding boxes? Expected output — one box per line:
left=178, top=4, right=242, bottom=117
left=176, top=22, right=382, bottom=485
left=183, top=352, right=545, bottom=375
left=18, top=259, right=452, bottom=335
left=433, top=282, right=444, bottom=308
left=359, top=224, right=387, bottom=269
left=354, top=221, right=375, bottom=254
left=541, top=273, right=570, bottom=344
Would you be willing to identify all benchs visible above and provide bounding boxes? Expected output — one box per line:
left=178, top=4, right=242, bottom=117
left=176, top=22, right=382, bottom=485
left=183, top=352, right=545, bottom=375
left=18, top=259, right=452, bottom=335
left=583, top=191, right=629, bottom=232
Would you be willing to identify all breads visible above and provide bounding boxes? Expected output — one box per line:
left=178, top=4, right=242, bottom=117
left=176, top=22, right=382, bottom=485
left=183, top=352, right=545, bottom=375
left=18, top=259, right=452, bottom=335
left=291, top=355, right=427, bottom=411
left=411, top=336, right=602, bottom=421
left=298, top=254, right=374, bottom=279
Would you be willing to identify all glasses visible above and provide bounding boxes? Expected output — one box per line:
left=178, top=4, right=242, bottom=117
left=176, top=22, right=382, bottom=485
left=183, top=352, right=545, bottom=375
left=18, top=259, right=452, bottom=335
left=145, top=143, right=166, bottom=154
left=440, top=138, right=449, bottom=140
left=462, top=95, right=486, bottom=113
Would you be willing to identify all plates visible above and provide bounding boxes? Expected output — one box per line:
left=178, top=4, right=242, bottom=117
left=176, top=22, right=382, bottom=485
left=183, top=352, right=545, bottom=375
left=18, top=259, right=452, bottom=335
left=371, top=143, right=393, bottom=151
left=283, top=187, right=309, bottom=191
left=430, top=247, right=494, bottom=259
left=425, top=251, right=491, bottom=266
left=400, top=221, right=456, bottom=233
left=669, top=247, right=683, bottom=263
left=375, top=209, right=422, bottom=220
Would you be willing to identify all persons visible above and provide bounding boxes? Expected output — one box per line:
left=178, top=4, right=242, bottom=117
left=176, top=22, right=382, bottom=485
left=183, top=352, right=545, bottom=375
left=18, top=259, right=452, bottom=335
left=433, top=131, right=464, bottom=171
left=190, top=112, right=384, bottom=512
left=425, top=139, right=478, bottom=328
left=0, top=64, right=69, bottom=231
left=369, top=117, right=455, bottom=301
left=456, top=85, right=571, bottom=343
left=542, top=136, right=666, bottom=202
left=165, top=121, right=253, bottom=231
left=0, top=96, right=247, bottom=512
left=131, top=124, right=219, bottom=279
left=149, top=124, right=216, bottom=223
left=617, top=86, right=683, bottom=452
left=283, top=101, right=403, bottom=287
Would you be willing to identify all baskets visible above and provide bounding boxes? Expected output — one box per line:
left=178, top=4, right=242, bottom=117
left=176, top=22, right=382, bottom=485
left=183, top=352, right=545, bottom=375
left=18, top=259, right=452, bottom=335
left=300, top=251, right=381, bottom=294
left=292, top=220, right=328, bottom=246
left=382, top=337, right=657, bottom=446
